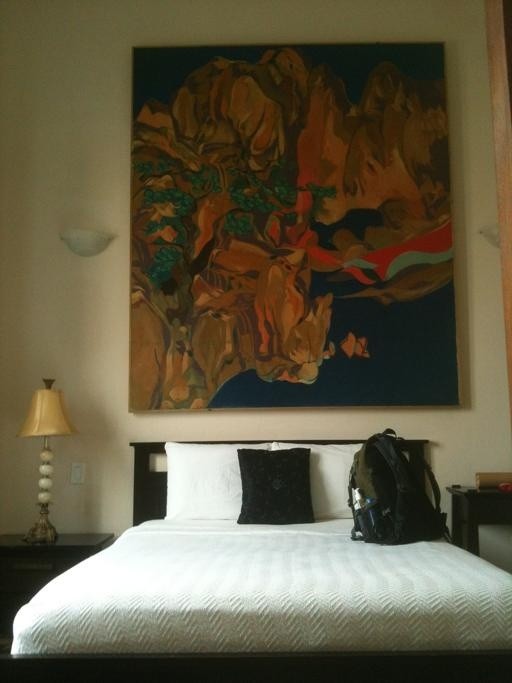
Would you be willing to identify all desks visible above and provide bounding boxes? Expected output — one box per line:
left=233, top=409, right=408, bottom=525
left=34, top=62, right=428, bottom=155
left=445, top=485, right=512, bottom=557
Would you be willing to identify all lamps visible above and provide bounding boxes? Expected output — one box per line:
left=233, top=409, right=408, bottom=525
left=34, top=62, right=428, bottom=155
left=19, top=377, right=80, bottom=543
left=478, top=223, right=500, bottom=249
left=58, top=229, right=116, bottom=257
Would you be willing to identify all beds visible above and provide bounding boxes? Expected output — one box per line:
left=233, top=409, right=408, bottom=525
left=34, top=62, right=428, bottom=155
left=0, top=439, right=512, bottom=683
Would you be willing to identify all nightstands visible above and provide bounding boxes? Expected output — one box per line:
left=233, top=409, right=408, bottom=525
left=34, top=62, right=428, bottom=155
left=0, top=533, right=115, bottom=636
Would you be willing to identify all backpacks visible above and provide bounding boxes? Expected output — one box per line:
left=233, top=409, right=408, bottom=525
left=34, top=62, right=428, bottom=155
left=348, top=429, right=446, bottom=544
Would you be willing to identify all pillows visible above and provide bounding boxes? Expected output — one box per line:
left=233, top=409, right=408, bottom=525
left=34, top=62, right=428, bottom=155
left=163, top=442, right=272, bottom=520
left=237, top=448, right=315, bottom=525
left=272, top=441, right=364, bottom=519
